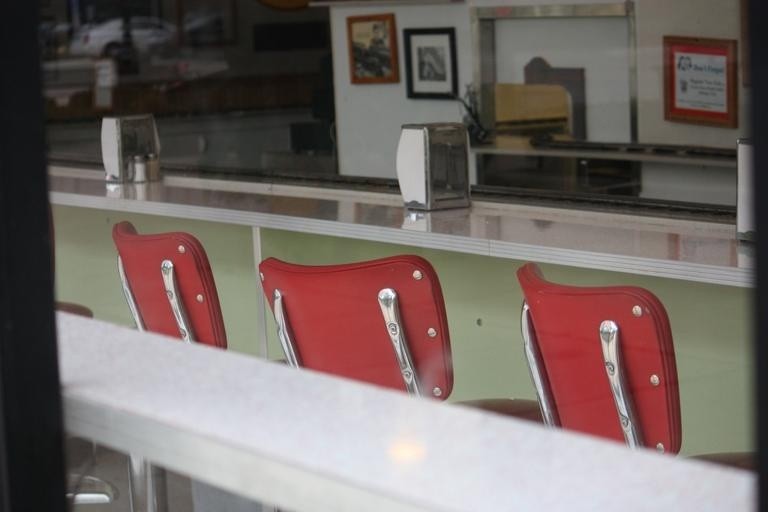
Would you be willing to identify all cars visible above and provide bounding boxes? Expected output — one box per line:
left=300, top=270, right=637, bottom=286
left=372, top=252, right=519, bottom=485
left=51, top=15, right=222, bottom=60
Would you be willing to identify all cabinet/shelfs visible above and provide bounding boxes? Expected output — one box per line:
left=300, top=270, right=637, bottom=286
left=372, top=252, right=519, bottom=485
left=470, top=137, right=739, bottom=220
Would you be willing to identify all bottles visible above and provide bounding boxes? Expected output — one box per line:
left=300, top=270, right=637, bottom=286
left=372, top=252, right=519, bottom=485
left=134, top=153, right=159, bottom=183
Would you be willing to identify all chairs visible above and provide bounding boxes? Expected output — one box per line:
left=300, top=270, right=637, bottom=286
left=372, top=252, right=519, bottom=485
left=259, top=255, right=542, bottom=424
left=109, top=219, right=226, bottom=351
left=517, top=261, right=754, bottom=471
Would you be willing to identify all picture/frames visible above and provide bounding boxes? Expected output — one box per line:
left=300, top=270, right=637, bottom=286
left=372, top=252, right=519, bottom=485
left=662, top=37, right=741, bottom=129
left=344, top=15, right=457, bottom=99
